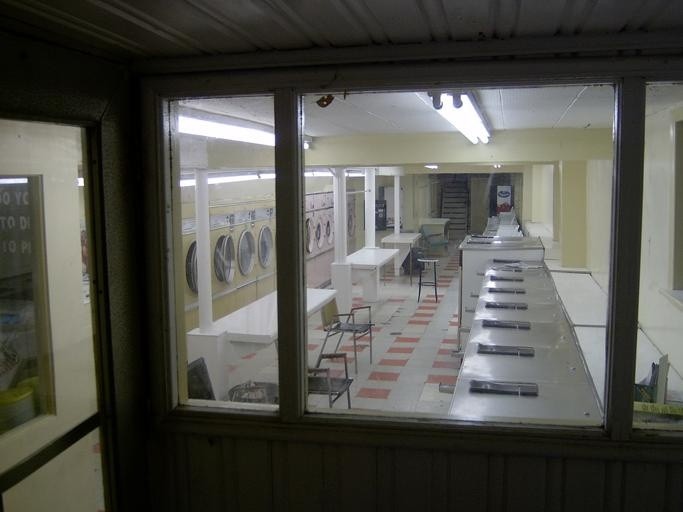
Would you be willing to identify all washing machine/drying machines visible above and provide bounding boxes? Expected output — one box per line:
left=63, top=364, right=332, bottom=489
left=180, top=175, right=356, bottom=371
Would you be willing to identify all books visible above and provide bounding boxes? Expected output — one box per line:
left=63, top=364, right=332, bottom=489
left=632, top=354, right=683, bottom=431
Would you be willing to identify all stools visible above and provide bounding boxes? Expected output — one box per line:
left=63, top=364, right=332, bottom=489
left=306, top=305, right=375, bottom=410
left=417, top=258, right=439, bottom=303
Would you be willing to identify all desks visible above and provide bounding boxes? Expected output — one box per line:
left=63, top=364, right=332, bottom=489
left=421, top=218, right=450, bottom=257
left=187, top=289, right=340, bottom=402
left=448, top=211, right=606, bottom=430
left=383, top=233, right=422, bottom=281
left=347, top=248, right=400, bottom=303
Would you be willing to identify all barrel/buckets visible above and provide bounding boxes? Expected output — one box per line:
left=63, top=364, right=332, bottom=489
left=1, top=385, right=35, bottom=432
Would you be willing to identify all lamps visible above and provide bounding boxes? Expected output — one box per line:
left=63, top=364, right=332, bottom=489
left=426, top=89, right=490, bottom=145
